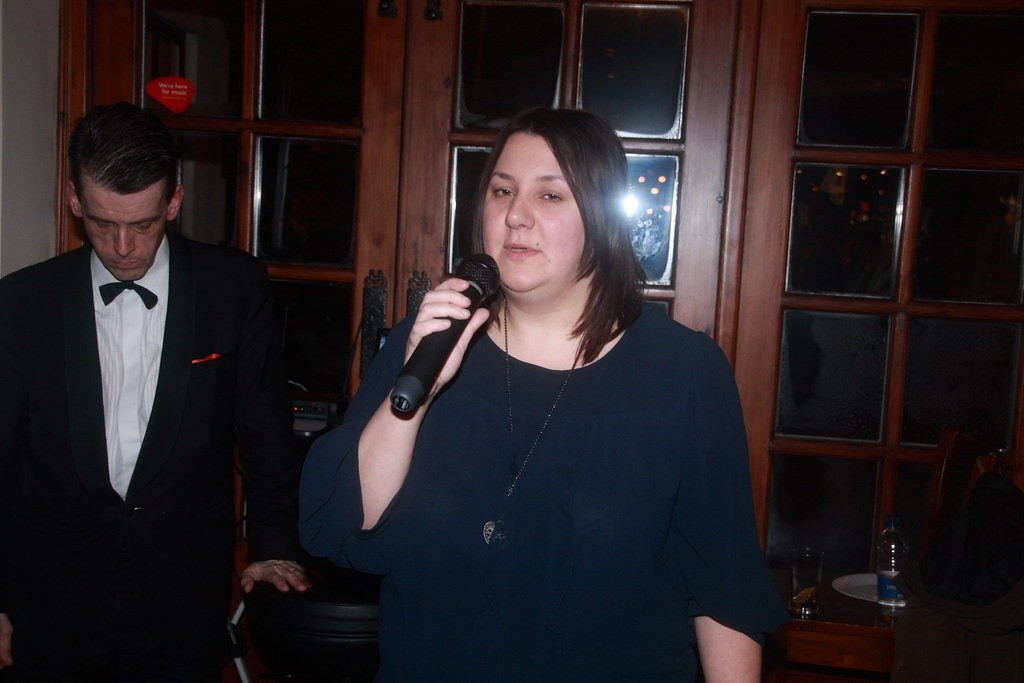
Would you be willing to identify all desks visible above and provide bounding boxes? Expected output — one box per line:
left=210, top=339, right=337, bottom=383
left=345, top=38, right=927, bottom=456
left=228, top=559, right=383, bottom=683
left=769, top=562, right=896, bottom=683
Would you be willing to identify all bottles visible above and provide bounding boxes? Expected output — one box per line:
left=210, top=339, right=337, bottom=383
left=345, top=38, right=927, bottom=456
left=876, top=515, right=909, bottom=617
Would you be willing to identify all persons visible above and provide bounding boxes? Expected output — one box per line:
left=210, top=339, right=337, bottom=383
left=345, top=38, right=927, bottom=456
left=0, top=100, right=325, bottom=683
left=298, top=110, right=793, bottom=683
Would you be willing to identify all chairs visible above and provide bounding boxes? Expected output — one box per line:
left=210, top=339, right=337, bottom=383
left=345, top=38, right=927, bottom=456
left=920, top=423, right=1014, bottom=551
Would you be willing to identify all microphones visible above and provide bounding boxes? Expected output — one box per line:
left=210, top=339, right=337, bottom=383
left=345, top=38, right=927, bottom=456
left=389, top=253, right=499, bottom=413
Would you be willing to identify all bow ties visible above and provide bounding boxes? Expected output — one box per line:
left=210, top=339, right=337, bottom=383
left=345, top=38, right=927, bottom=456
left=99, top=276, right=158, bottom=311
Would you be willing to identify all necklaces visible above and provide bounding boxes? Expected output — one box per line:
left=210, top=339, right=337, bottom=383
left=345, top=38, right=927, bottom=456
left=483, top=299, right=586, bottom=548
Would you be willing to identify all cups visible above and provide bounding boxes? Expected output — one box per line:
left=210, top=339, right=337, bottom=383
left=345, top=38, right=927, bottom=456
left=788, top=546, right=825, bottom=616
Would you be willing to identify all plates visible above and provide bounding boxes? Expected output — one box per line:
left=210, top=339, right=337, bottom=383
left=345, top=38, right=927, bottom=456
left=832, top=572, right=878, bottom=603
